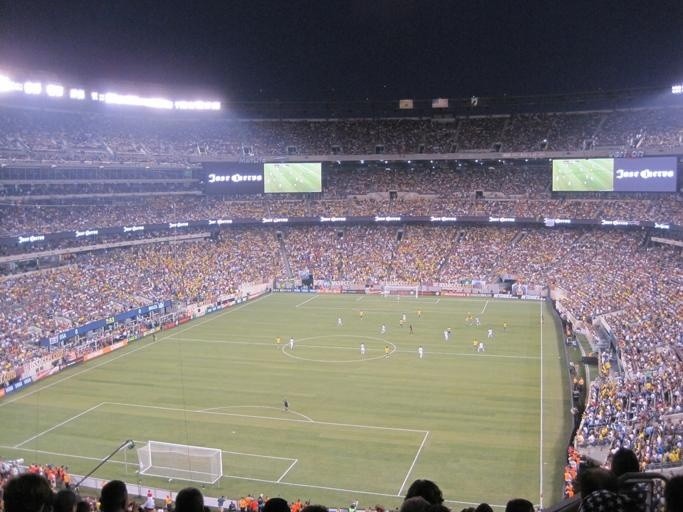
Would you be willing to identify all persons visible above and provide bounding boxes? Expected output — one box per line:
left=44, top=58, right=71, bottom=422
left=2, top=109, right=683, bottom=512
